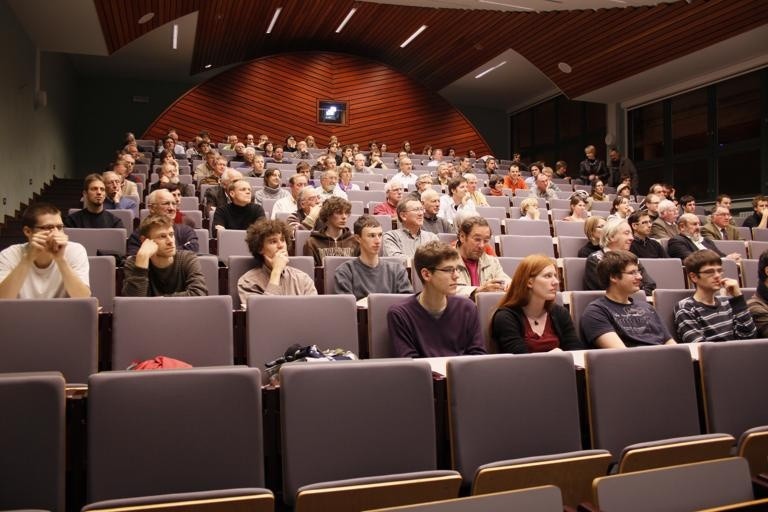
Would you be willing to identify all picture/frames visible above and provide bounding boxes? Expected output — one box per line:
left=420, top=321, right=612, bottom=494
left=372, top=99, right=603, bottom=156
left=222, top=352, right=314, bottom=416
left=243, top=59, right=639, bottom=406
left=316, top=99, right=350, bottom=127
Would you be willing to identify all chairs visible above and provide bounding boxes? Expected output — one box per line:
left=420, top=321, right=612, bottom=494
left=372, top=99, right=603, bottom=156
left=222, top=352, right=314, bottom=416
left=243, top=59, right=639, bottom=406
left=0, top=140, right=768, bottom=512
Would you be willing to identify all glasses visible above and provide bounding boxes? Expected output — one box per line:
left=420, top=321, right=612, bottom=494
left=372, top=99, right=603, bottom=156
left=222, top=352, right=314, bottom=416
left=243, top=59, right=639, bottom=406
left=699, top=268, right=725, bottom=276
left=405, top=207, right=426, bottom=213
left=621, top=270, right=642, bottom=275
left=35, top=225, right=65, bottom=230
left=433, top=266, right=461, bottom=274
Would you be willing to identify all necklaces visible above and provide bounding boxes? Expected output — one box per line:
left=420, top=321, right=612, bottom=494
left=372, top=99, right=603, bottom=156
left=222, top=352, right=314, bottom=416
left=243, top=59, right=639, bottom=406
left=524, top=311, right=544, bottom=325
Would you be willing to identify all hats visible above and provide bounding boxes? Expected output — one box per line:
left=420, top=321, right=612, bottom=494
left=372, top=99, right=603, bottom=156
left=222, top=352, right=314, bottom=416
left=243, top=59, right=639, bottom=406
left=616, top=183, right=630, bottom=195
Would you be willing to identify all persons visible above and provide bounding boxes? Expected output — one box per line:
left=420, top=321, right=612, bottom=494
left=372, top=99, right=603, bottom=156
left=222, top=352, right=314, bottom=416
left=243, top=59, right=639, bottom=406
left=121, top=215, right=210, bottom=296
left=1, top=203, right=92, bottom=299
left=670, top=250, right=756, bottom=342
left=452, top=216, right=513, bottom=300
left=238, top=217, right=318, bottom=312
left=746, top=251, right=768, bottom=338
left=302, top=136, right=768, bottom=268
left=579, top=248, right=678, bottom=351
left=386, top=242, right=482, bottom=354
left=65, top=131, right=324, bottom=240
left=332, top=215, right=414, bottom=310
left=484, top=256, right=583, bottom=353
